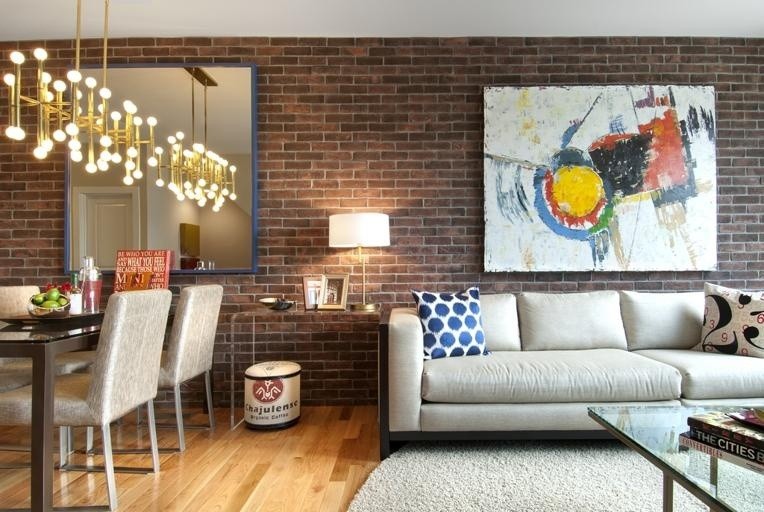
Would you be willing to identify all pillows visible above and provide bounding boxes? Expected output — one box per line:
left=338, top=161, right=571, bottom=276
left=408, top=282, right=492, bottom=360
left=690, top=280, right=764, bottom=358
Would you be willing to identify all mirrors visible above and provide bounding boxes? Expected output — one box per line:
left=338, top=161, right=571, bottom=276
left=61, top=60, right=259, bottom=278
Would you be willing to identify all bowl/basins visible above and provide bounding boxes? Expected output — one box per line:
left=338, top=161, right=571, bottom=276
left=260, top=297, right=293, bottom=310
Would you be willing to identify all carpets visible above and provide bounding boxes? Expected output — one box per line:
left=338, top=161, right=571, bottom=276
left=342, top=435, right=764, bottom=512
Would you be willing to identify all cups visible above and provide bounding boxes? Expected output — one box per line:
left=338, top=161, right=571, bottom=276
left=195, top=255, right=216, bottom=270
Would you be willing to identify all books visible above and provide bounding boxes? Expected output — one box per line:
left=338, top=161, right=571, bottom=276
left=677, top=405, right=763, bottom=475
left=113, top=248, right=170, bottom=292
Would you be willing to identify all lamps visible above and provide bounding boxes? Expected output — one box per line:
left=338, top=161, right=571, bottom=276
left=1, top=0, right=157, bottom=185
left=328, top=212, right=391, bottom=313
left=148, top=62, right=236, bottom=211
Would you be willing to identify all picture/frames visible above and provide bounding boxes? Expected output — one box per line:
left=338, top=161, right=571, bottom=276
left=302, top=273, right=350, bottom=312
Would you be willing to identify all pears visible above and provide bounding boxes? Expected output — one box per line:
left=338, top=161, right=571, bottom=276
left=32, top=288, right=67, bottom=308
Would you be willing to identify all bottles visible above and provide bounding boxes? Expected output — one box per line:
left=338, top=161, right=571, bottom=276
left=68, top=270, right=82, bottom=315
left=78, top=254, right=102, bottom=313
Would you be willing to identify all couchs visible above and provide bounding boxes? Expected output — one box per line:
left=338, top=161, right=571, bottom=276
left=375, top=288, right=764, bottom=462
